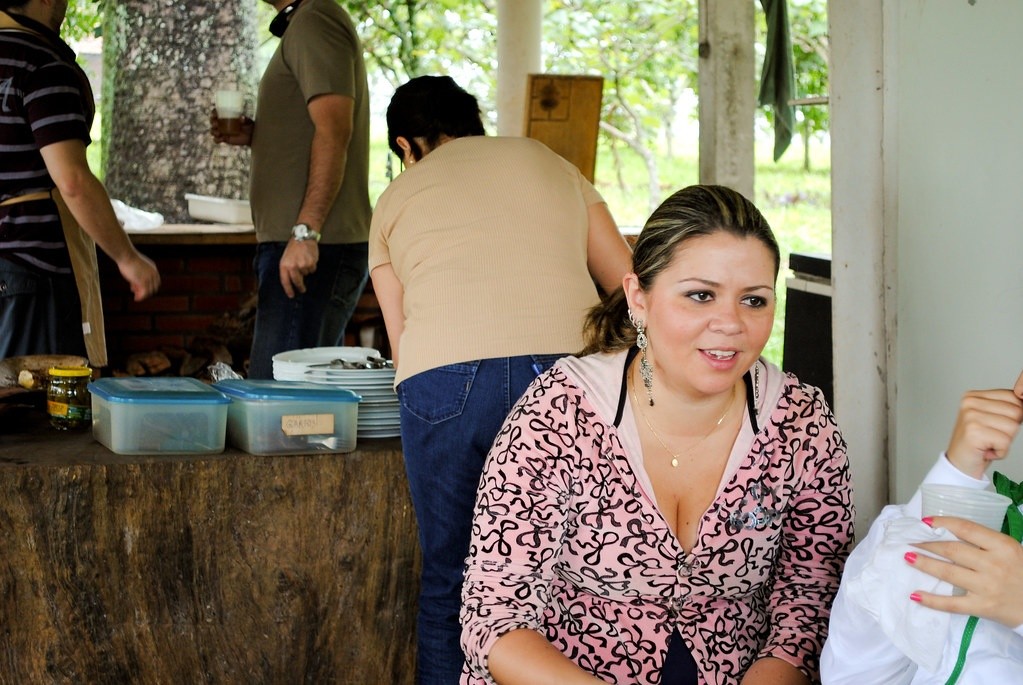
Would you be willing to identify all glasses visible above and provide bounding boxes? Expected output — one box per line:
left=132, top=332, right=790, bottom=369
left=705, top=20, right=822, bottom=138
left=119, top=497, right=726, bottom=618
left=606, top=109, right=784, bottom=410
left=270, top=0, right=304, bottom=37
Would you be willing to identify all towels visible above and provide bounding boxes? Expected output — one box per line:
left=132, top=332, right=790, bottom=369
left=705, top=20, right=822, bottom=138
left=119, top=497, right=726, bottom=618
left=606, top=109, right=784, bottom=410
left=757, top=1, right=798, bottom=163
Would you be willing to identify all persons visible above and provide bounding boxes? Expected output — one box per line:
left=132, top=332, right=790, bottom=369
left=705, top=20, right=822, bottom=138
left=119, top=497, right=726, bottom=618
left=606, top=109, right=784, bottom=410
left=369, top=75, right=633, bottom=685
left=0, top=0, right=160, bottom=364
left=820, top=372, right=1023, bottom=684
left=209, top=0, right=373, bottom=382
left=457, top=183, right=853, bottom=685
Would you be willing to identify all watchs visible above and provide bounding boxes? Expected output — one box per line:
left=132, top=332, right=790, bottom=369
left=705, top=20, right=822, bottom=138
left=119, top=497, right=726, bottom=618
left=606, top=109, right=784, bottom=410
left=290, top=224, right=318, bottom=240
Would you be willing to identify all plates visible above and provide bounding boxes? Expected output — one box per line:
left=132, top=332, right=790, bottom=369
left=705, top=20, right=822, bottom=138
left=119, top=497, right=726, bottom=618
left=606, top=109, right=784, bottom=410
left=273, top=347, right=402, bottom=439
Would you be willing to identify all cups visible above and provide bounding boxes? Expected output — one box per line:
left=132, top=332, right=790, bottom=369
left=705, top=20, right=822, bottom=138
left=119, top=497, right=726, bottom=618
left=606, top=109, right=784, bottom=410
left=921, top=484, right=1012, bottom=596
left=214, top=91, right=245, bottom=135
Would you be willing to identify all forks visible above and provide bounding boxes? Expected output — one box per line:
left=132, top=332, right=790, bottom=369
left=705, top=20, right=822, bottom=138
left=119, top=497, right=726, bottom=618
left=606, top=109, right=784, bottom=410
left=307, top=436, right=351, bottom=450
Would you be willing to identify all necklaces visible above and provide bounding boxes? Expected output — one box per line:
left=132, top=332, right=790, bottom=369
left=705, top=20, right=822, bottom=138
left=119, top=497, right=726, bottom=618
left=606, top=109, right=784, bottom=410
left=632, top=361, right=735, bottom=467
